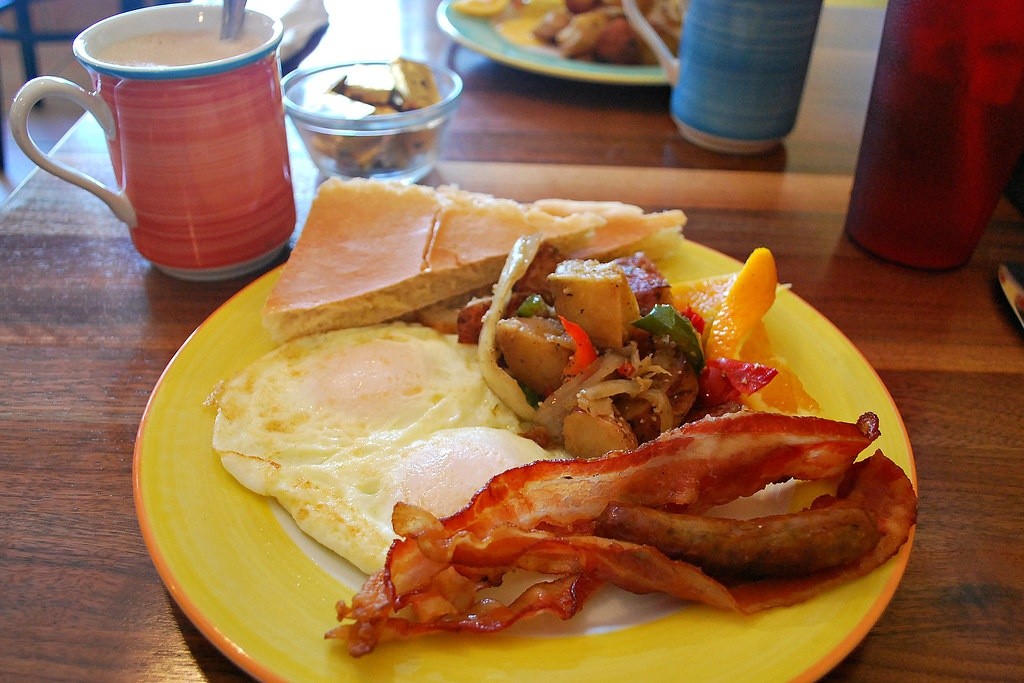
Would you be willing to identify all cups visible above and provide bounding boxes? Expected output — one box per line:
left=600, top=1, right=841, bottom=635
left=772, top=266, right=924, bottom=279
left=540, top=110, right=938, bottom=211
left=620, top=0, right=827, bottom=154
left=844, top=0, right=1024, bottom=271
left=12, top=3, right=296, bottom=288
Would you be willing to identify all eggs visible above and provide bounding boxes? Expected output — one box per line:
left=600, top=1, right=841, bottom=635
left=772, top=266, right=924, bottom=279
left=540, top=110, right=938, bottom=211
left=205, top=321, right=530, bottom=496
left=267, top=427, right=560, bottom=577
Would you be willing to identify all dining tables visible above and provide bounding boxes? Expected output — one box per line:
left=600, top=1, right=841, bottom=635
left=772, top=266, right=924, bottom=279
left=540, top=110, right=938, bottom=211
left=0, top=0, right=1022, bottom=683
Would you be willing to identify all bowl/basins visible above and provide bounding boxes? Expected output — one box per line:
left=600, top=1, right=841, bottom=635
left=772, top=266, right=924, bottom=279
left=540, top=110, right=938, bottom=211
left=282, top=59, right=463, bottom=186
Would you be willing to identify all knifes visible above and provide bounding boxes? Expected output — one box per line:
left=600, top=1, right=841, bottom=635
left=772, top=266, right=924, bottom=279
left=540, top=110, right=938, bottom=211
left=996, top=264, right=1023, bottom=329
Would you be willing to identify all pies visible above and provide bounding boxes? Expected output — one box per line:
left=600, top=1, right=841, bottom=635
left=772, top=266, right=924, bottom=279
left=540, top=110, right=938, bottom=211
left=535, top=198, right=688, bottom=260
left=265, top=175, right=608, bottom=343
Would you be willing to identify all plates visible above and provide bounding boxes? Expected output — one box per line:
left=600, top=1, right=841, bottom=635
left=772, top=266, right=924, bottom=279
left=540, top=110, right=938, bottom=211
left=437, top=0, right=679, bottom=87
left=133, top=228, right=918, bottom=681
